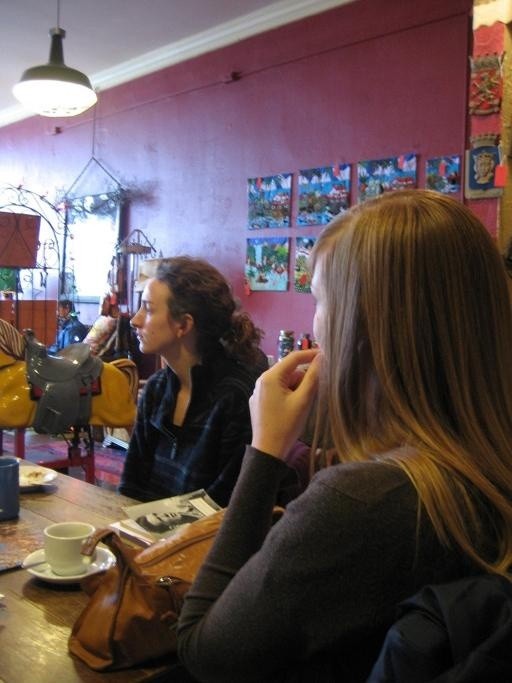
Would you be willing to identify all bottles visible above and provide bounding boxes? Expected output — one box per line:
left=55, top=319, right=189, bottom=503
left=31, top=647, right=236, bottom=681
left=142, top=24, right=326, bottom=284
left=278, top=328, right=312, bottom=365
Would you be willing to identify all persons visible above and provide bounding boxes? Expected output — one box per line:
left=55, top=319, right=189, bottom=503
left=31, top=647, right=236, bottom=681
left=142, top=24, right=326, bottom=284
left=175, top=188, right=511, bottom=680
left=46, top=301, right=85, bottom=355
left=474, top=152, right=494, bottom=184
left=118, top=256, right=254, bottom=508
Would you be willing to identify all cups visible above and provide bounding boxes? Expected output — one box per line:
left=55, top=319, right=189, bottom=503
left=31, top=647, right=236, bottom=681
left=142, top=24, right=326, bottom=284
left=42, top=522, right=97, bottom=575
left=0, top=457, right=20, bottom=522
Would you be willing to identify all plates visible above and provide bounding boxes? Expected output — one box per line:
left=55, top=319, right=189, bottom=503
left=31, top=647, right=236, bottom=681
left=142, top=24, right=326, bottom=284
left=20, top=466, right=58, bottom=492
left=23, top=547, right=116, bottom=586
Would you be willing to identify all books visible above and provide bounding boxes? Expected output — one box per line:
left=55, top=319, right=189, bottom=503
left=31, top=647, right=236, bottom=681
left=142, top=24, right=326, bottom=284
left=109, top=488, right=224, bottom=548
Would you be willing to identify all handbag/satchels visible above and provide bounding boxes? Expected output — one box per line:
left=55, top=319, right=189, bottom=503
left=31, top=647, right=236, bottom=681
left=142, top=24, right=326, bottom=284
left=68, top=510, right=226, bottom=681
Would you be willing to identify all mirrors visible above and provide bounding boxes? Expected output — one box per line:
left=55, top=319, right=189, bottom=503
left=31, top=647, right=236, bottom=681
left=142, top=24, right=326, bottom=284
left=59, top=189, right=124, bottom=302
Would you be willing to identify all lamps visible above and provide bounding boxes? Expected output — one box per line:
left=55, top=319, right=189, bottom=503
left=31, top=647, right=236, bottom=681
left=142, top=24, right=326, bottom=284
left=11, top=0, right=97, bottom=117
